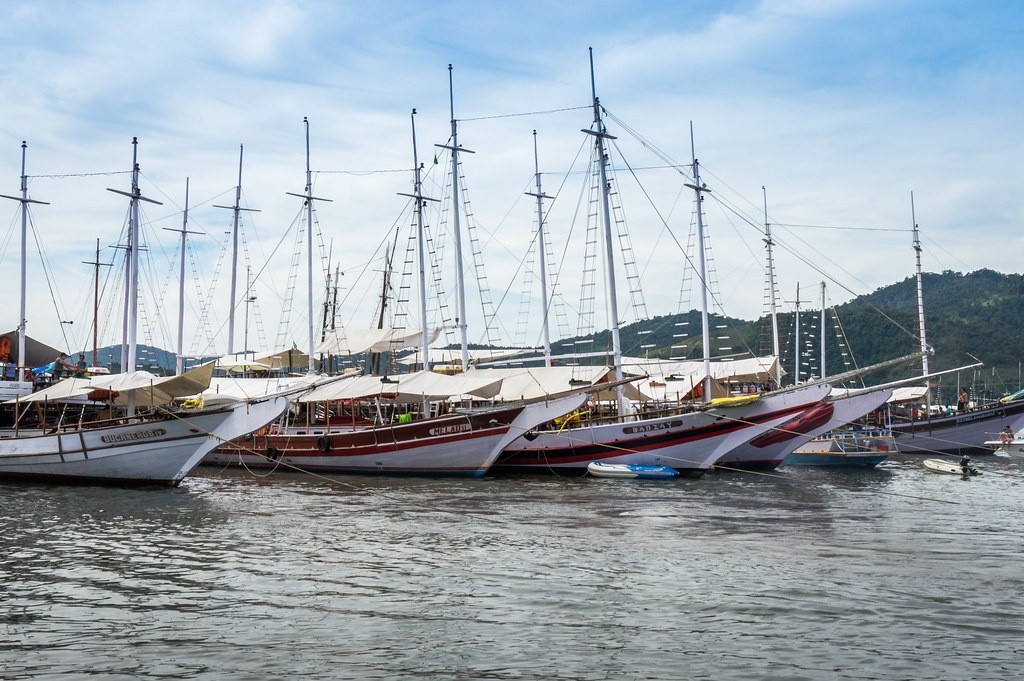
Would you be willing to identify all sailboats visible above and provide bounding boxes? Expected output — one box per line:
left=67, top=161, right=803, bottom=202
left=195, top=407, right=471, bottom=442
left=0, top=47, right=1024, bottom=489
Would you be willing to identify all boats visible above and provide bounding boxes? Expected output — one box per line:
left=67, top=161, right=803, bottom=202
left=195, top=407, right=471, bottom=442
left=923, top=455, right=982, bottom=476
left=587, top=460, right=679, bottom=480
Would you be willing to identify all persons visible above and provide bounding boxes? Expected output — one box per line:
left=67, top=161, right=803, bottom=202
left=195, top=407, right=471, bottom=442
left=958, top=388, right=968, bottom=414
left=75, top=354, right=87, bottom=377
left=50, top=352, right=78, bottom=381
left=911, top=403, right=919, bottom=422
left=945, top=404, right=954, bottom=417
left=998, top=425, right=1014, bottom=453
left=33, top=372, right=39, bottom=387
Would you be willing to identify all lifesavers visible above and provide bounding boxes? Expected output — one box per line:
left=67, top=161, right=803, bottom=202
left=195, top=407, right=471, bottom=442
left=316, top=435, right=331, bottom=452
left=265, top=447, right=278, bottom=462
left=243, top=432, right=253, bottom=442
